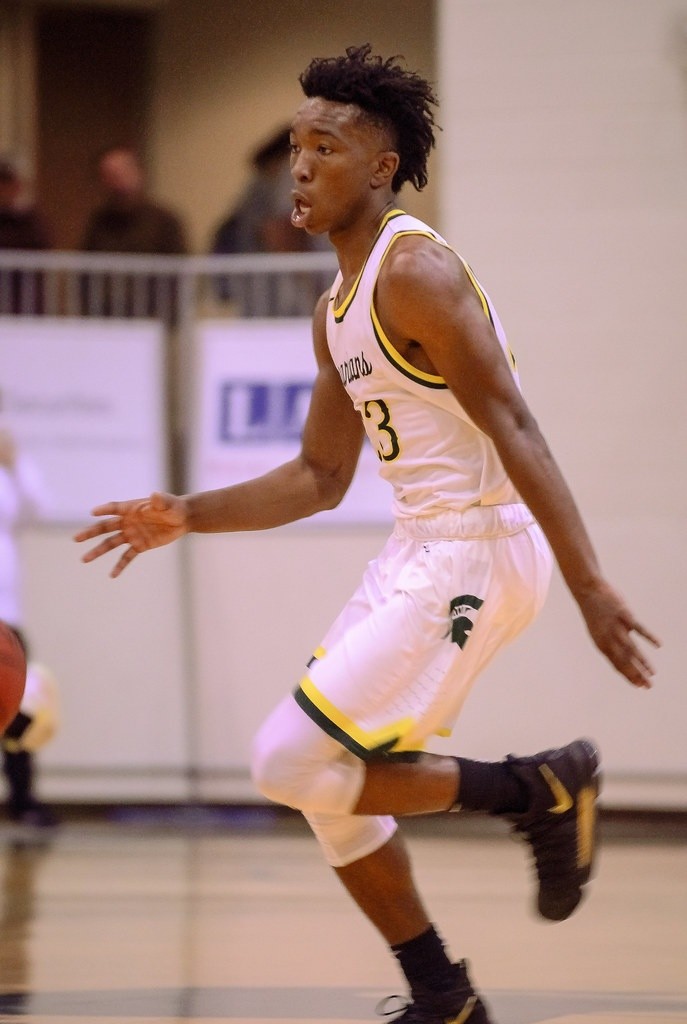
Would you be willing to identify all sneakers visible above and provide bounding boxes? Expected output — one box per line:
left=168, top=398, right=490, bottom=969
left=508, top=736, right=599, bottom=922
left=391, top=962, right=493, bottom=1024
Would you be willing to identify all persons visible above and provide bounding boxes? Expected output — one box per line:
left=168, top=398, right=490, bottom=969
left=74, top=148, right=189, bottom=318
left=0, top=161, right=52, bottom=316
left=209, top=120, right=340, bottom=316
left=0, top=401, right=60, bottom=832
left=78, top=45, right=659, bottom=1024
